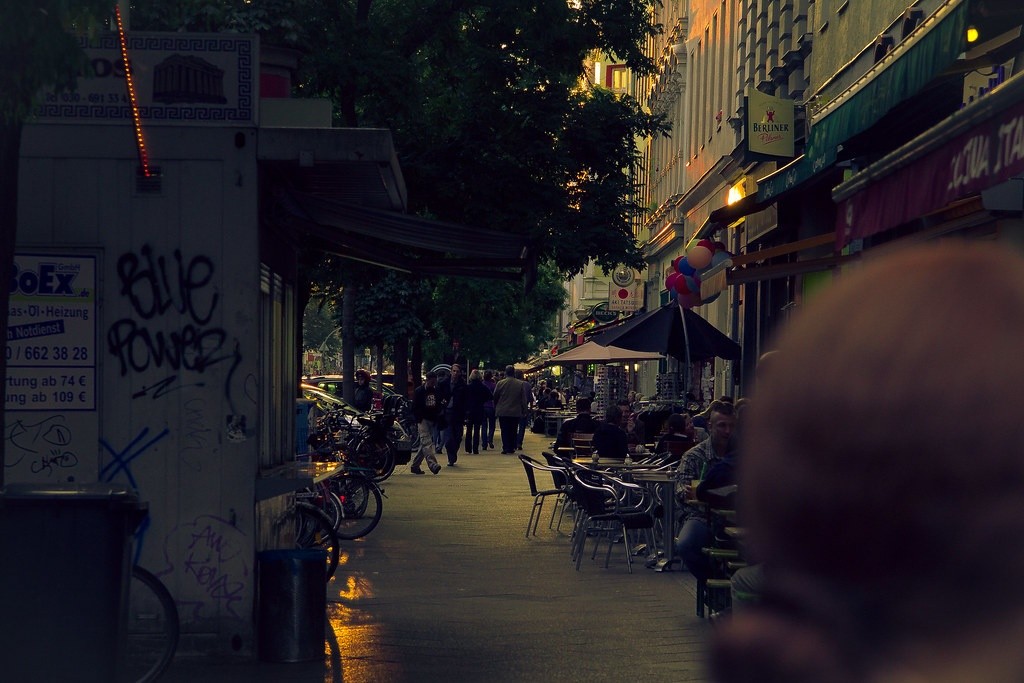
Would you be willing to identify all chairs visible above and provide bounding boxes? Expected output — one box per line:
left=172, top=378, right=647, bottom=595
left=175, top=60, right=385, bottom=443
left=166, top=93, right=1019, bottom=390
left=519, top=406, right=745, bottom=619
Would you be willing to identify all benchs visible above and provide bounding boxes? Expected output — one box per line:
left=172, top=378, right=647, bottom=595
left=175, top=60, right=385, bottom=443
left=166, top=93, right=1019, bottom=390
left=701, top=507, right=756, bottom=617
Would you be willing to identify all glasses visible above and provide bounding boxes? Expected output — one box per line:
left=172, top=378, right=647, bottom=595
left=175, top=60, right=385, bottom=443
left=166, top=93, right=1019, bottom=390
left=656, top=374, right=680, bottom=400
left=616, top=366, right=629, bottom=404
left=597, top=367, right=608, bottom=422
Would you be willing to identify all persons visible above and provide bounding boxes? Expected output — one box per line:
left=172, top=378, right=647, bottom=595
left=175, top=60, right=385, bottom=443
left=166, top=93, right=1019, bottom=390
left=465, top=366, right=533, bottom=453
left=410, top=364, right=466, bottom=475
left=353, top=369, right=376, bottom=411
left=674, top=402, right=779, bottom=628
left=708, top=239, right=1024, bottom=683
left=528, top=376, right=751, bottom=485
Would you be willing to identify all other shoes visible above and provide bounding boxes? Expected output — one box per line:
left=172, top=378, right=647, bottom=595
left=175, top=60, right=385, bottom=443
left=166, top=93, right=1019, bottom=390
left=501, top=450, right=509, bottom=454
left=411, top=468, right=426, bottom=475
left=431, top=465, right=441, bottom=475
left=482, top=447, right=487, bottom=450
left=447, top=462, right=454, bottom=466
left=489, top=443, right=494, bottom=449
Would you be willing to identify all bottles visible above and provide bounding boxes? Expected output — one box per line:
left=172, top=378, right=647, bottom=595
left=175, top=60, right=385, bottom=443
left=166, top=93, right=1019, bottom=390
left=666, top=471, right=673, bottom=480
left=625, top=457, right=632, bottom=466
left=592, top=452, right=599, bottom=462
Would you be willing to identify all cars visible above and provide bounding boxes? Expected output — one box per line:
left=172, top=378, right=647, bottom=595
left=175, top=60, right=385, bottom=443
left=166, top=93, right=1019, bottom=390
left=297, top=366, right=429, bottom=584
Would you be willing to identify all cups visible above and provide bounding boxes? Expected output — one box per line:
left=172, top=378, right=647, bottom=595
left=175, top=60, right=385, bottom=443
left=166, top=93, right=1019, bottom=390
left=692, top=480, right=703, bottom=500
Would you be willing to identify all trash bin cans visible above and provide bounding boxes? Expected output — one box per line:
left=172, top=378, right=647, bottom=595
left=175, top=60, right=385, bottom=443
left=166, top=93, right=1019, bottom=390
left=0, top=482, right=138, bottom=683
left=257, top=548, right=327, bottom=663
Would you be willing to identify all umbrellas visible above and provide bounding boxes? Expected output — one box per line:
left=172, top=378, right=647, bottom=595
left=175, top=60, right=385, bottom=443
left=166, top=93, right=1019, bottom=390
left=506, top=362, right=535, bottom=369
left=587, top=300, right=742, bottom=400
left=548, top=341, right=667, bottom=361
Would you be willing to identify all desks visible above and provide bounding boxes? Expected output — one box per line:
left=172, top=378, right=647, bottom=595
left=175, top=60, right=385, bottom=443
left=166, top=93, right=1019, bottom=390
left=629, top=452, right=655, bottom=458
left=631, top=472, right=672, bottom=565
left=617, top=470, right=664, bottom=554
left=634, top=476, right=681, bottom=571
left=599, top=463, right=659, bottom=505
left=572, top=457, right=622, bottom=466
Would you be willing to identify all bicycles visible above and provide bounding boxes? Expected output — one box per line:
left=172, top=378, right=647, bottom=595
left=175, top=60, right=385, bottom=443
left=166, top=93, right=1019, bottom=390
left=120, top=564, right=182, bottom=683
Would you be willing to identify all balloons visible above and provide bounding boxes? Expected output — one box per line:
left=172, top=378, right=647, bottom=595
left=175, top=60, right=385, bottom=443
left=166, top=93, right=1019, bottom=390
left=664, top=238, right=734, bottom=308
